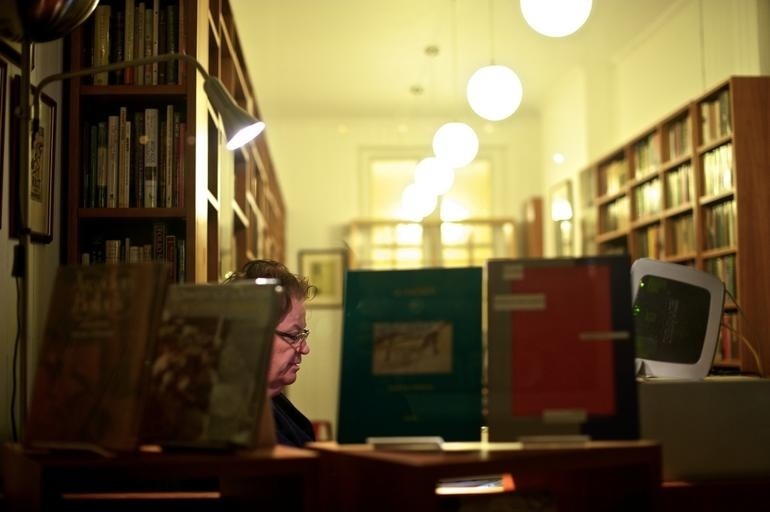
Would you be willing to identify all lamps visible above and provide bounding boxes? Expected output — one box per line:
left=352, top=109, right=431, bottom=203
left=37, top=50, right=268, bottom=154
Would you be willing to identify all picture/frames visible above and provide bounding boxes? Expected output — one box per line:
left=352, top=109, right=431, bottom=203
left=579, top=165, right=594, bottom=255
left=296, top=248, right=347, bottom=309
left=11, top=74, right=57, bottom=244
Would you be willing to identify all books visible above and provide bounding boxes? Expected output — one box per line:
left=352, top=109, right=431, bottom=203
left=601, top=88, right=734, bottom=362
left=80, top=0, right=186, bottom=286
left=334, top=254, right=643, bottom=446
left=24, top=258, right=276, bottom=457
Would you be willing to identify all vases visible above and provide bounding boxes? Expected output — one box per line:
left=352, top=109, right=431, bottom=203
left=549, top=181, right=573, bottom=257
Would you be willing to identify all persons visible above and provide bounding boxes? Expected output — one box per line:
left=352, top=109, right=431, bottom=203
left=224, top=258, right=318, bottom=448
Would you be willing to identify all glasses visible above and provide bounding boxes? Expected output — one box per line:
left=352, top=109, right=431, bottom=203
left=274, top=329, right=312, bottom=348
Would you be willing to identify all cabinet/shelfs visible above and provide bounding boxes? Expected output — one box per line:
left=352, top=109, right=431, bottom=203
left=61, top=1, right=288, bottom=285
left=592, top=76, right=768, bottom=374
